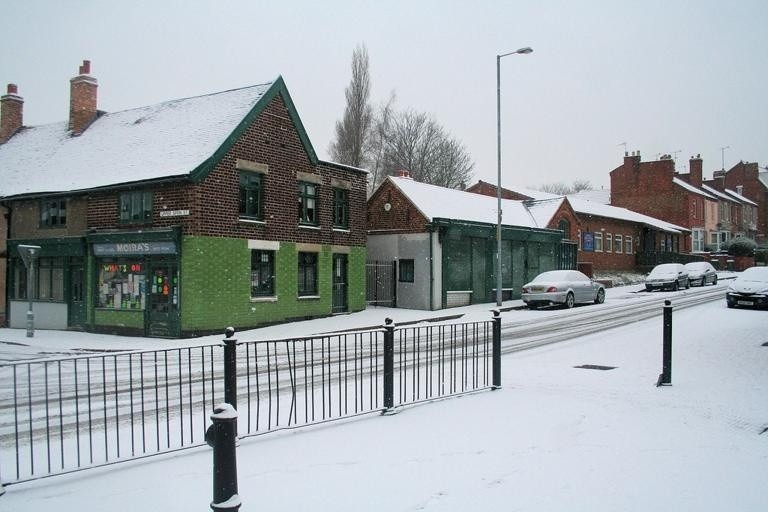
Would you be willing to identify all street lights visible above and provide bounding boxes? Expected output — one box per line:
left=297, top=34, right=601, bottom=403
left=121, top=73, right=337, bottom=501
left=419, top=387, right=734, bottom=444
left=496, top=48, right=533, bottom=307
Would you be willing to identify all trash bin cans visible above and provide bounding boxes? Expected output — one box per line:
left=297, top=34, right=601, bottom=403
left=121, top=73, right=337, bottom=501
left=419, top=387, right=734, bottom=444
left=727, top=260, right=734, bottom=271
left=710, top=259, right=719, bottom=271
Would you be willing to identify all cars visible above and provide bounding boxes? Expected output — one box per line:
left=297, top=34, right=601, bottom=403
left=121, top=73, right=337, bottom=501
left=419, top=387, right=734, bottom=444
left=726, top=267, right=768, bottom=307
left=521, top=270, right=605, bottom=308
left=645, top=262, right=717, bottom=292
